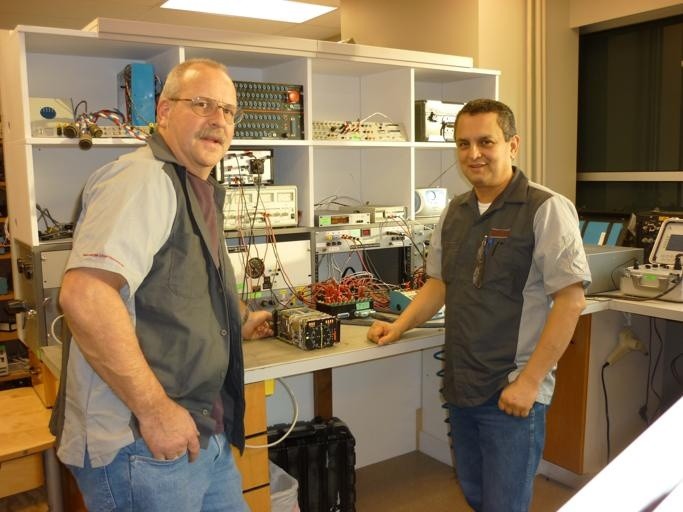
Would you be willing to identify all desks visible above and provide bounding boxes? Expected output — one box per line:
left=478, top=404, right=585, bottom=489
left=29, top=288, right=682, bottom=510
left=1, top=384, right=75, bottom=510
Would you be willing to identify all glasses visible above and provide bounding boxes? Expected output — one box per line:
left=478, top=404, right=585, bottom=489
left=169, top=96, right=244, bottom=125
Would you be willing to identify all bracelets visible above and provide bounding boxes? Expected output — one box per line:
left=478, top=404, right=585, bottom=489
left=240, top=306, right=250, bottom=328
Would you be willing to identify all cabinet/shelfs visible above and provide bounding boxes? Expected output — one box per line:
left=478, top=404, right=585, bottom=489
left=1, top=24, right=502, bottom=250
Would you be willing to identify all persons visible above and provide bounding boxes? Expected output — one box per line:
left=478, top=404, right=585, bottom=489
left=366, top=98, right=593, bottom=512
left=50, top=56, right=274, bottom=512
